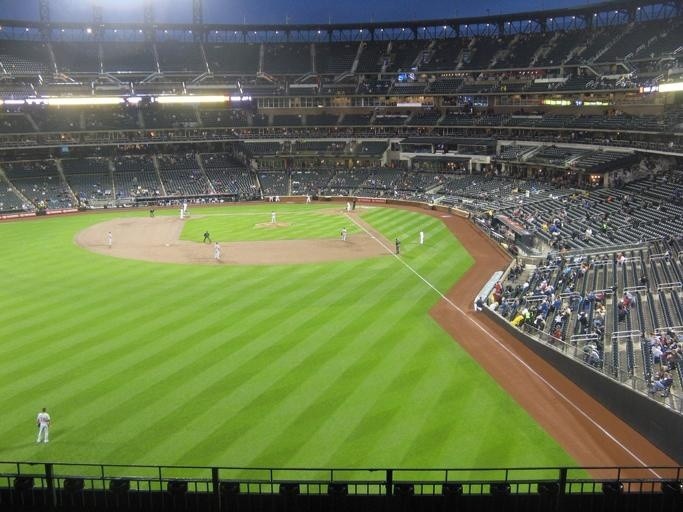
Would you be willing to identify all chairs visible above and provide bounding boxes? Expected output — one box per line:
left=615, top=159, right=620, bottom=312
left=484, top=19, right=681, bottom=410
left=2, top=38, right=482, bottom=227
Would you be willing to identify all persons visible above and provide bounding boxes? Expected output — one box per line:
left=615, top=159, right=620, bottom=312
left=179, top=206, right=184, bottom=220
left=107, top=232, right=112, bottom=247
left=202, top=230, right=211, bottom=244
left=270, top=211, right=276, bottom=224
left=418, top=230, right=424, bottom=244
left=467, top=214, right=683, bottom=398
left=149, top=208, right=154, bottom=218
left=213, top=241, right=221, bottom=259
left=340, top=226, right=346, bottom=241
left=36, top=407, right=51, bottom=444
left=1, top=69, right=683, bottom=214
left=395, top=238, right=401, bottom=254
